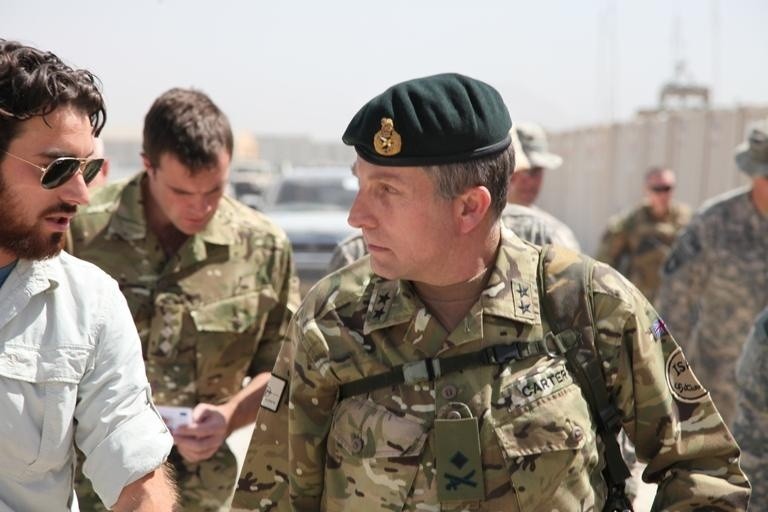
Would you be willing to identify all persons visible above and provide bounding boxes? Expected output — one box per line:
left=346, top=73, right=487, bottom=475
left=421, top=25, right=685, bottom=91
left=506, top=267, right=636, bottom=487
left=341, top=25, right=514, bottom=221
left=641, top=117, right=768, bottom=510
left=587, top=166, right=693, bottom=305
left=0, top=36, right=182, bottom=511
left=498, top=115, right=564, bottom=208
left=323, top=125, right=563, bottom=280
left=54, top=86, right=307, bottom=511
left=216, top=78, right=755, bottom=512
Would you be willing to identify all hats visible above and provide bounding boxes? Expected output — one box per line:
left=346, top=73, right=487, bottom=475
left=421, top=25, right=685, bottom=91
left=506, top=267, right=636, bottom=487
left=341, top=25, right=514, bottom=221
left=734, top=119, right=768, bottom=183
left=508, top=118, right=563, bottom=173
left=341, top=71, right=512, bottom=168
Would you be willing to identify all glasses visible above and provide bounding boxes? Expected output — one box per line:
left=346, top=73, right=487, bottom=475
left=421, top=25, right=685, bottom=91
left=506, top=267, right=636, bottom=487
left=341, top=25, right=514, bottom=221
left=650, top=185, right=674, bottom=192
left=1, top=146, right=109, bottom=191
